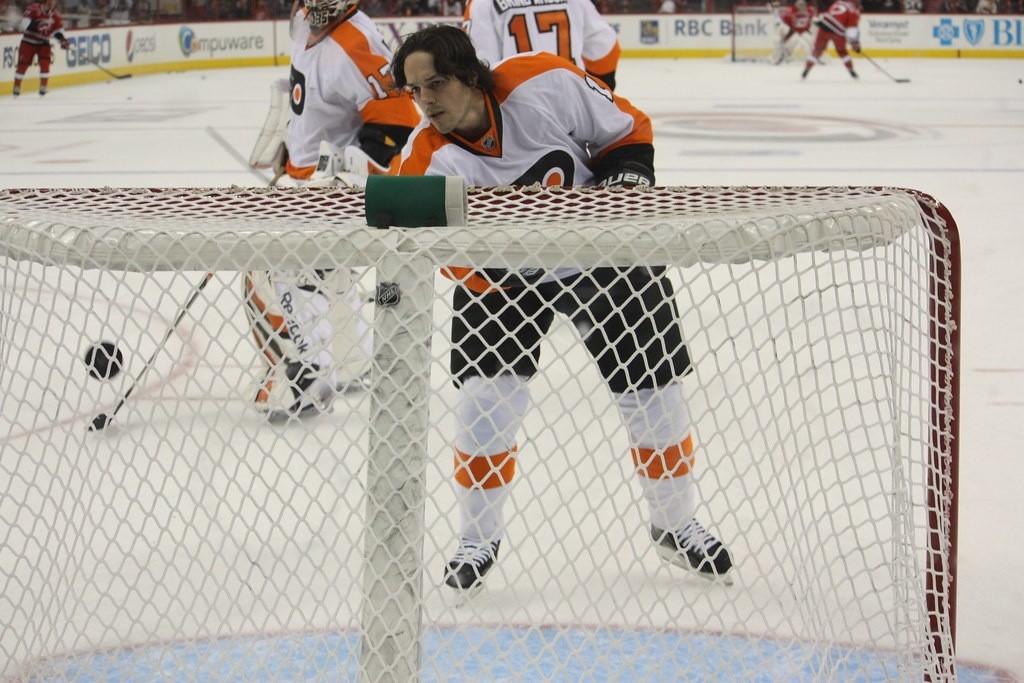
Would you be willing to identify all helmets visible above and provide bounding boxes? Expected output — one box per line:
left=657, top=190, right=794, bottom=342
left=303, top=0, right=357, bottom=36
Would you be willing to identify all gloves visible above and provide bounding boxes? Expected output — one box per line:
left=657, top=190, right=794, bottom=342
left=851, top=42, right=860, bottom=53
left=55, top=32, right=70, bottom=49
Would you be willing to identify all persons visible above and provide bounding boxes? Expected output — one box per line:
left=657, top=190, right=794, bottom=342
left=13, top=0, right=69, bottom=94
left=457, top=0, right=620, bottom=91
left=243, top=0, right=423, bottom=420
left=769, top=0, right=860, bottom=78
left=388, top=25, right=738, bottom=606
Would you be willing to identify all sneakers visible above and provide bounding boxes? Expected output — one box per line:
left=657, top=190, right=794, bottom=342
left=39, top=85, right=46, bottom=95
left=14, top=86, right=20, bottom=94
left=652, top=516, right=734, bottom=585
left=255, top=357, right=339, bottom=423
left=444, top=531, right=505, bottom=608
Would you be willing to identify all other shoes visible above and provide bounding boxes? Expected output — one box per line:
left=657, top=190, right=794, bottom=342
left=802, top=67, right=809, bottom=77
left=850, top=69, right=857, bottom=77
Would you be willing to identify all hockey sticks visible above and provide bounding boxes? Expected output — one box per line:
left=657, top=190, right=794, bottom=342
left=859, top=49, right=910, bottom=82
left=67, top=46, right=132, bottom=80
left=83, top=167, right=285, bottom=433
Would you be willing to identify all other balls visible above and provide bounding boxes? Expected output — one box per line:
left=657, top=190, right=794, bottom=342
left=84, top=341, right=123, bottom=382
left=1018, top=79, right=1022, bottom=83
